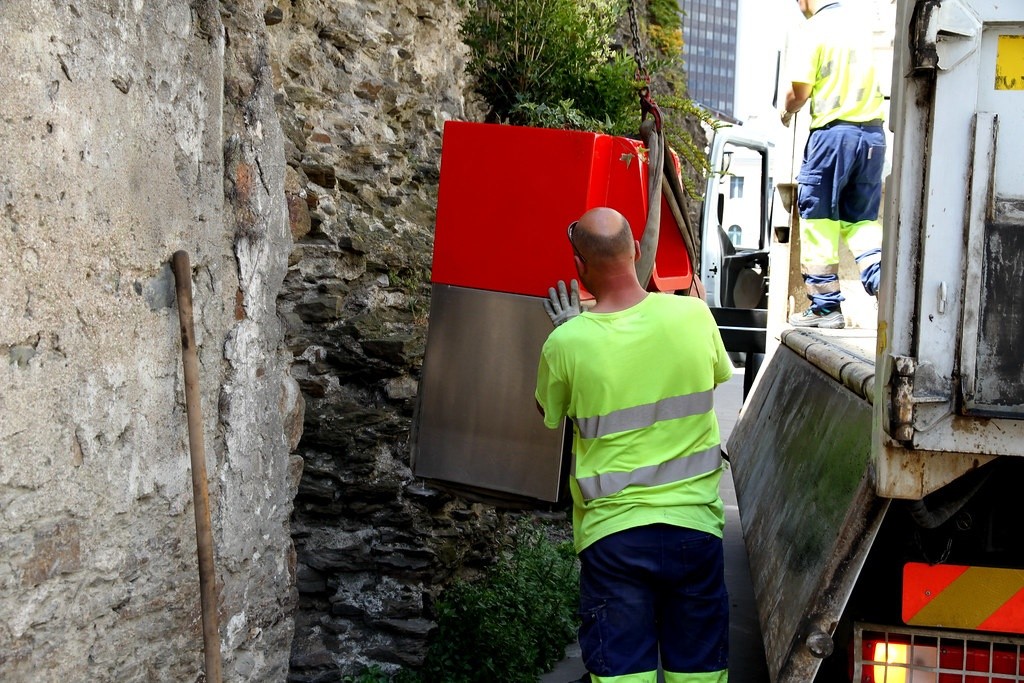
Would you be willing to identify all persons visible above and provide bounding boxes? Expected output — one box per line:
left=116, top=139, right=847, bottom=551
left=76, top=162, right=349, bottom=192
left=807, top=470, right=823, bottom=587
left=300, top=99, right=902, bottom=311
left=534, top=207, right=735, bottom=683
left=781, top=0, right=883, bottom=329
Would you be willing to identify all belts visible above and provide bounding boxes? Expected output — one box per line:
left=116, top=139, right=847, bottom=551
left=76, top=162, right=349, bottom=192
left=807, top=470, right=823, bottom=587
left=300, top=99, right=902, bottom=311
left=829, top=118, right=883, bottom=127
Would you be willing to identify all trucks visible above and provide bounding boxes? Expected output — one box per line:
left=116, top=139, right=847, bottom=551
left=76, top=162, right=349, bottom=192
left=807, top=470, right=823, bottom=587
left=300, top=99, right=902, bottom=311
left=700, top=1, right=1024, bottom=683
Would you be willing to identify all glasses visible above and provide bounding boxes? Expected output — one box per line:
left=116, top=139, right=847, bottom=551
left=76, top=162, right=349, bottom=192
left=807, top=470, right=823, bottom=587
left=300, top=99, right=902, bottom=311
left=567, top=221, right=586, bottom=263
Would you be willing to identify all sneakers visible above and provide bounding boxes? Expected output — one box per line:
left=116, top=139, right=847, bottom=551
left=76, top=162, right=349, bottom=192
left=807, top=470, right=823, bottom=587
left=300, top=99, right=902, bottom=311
left=789, top=306, right=845, bottom=329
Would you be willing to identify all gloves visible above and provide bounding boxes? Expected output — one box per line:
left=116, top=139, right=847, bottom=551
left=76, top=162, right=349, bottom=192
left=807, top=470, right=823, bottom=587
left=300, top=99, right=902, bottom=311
left=543, top=279, right=588, bottom=328
left=780, top=111, right=794, bottom=128
